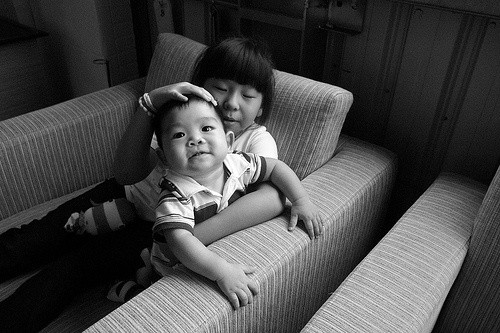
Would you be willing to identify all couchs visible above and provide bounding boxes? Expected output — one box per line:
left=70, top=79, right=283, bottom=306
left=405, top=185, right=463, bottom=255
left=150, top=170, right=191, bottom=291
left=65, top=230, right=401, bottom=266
left=0, top=32, right=399, bottom=333
left=300, top=164, right=500, bottom=333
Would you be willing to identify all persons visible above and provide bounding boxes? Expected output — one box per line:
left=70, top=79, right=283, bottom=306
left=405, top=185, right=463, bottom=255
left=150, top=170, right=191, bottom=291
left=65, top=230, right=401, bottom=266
left=106, top=91, right=325, bottom=310
left=0, top=36, right=286, bottom=333
